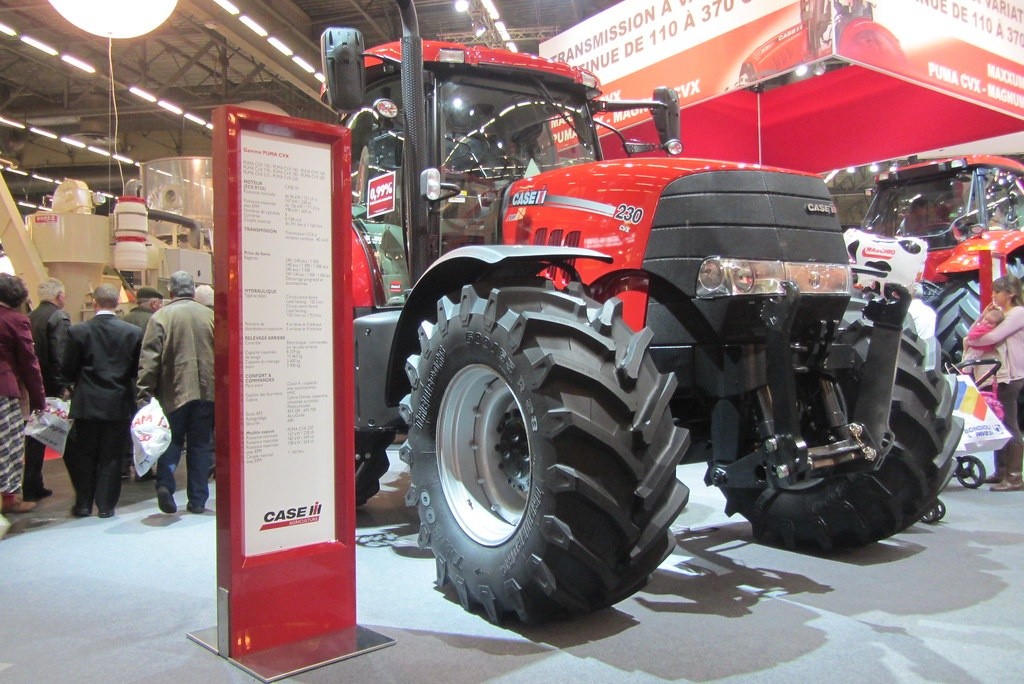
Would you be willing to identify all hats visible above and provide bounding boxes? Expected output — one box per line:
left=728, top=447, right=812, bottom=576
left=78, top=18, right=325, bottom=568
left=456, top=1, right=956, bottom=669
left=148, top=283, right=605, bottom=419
left=167, top=270, right=195, bottom=296
left=194, top=284, right=214, bottom=306
left=137, top=287, right=163, bottom=299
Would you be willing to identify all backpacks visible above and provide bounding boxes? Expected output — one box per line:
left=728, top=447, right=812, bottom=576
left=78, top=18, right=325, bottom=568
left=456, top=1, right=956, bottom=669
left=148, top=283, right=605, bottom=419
left=979, top=373, right=1004, bottom=421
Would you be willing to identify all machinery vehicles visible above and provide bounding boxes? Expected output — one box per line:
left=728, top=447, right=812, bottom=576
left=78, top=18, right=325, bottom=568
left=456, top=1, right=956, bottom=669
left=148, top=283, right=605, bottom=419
left=852, top=151, right=1024, bottom=374
left=320, top=0, right=967, bottom=632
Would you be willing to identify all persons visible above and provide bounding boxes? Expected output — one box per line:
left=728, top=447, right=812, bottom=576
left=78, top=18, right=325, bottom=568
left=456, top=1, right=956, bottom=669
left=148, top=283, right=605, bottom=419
left=961, top=310, right=1005, bottom=376
left=66, top=282, right=144, bottom=517
left=967, top=275, right=1024, bottom=491
left=22, top=277, right=76, bottom=502
left=896, top=196, right=930, bottom=237
left=908, top=284, right=939, bottom=385
left=194, top=284, right=215, bottom=477
left=119, top=287, right=164, bottom=482
left=0, top=272, right=47, bottom=514
left=136, top=271, right=215, bottom=513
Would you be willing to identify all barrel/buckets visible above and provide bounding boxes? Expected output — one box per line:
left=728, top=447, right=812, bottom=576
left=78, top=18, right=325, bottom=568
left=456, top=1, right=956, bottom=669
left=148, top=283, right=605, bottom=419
left=112, top=196, right=148, bottom=238
left=113, top=235, right=148, bottom=270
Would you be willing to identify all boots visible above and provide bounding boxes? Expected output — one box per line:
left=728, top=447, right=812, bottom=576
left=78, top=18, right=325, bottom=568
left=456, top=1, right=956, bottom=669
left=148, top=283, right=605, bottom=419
left=1, top=492, right=37, bottom=514
left=985, top=444, right=1024, bottom=490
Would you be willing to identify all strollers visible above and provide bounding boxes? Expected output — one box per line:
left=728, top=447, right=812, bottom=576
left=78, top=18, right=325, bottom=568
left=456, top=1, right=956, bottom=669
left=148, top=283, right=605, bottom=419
left=940, top=358, right=1001, bottom=491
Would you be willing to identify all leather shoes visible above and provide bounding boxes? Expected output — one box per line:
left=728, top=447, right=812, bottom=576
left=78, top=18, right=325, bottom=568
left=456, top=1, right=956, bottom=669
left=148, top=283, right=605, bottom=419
left=23, top=488, right=53, bottom=501
left=97, top=503, right=115, bottom=518
left=156, top=486, right=177, bottom=513
left=187, top=501, right=205, bottom=514
left=71, top=496, right=94, bottom=518
